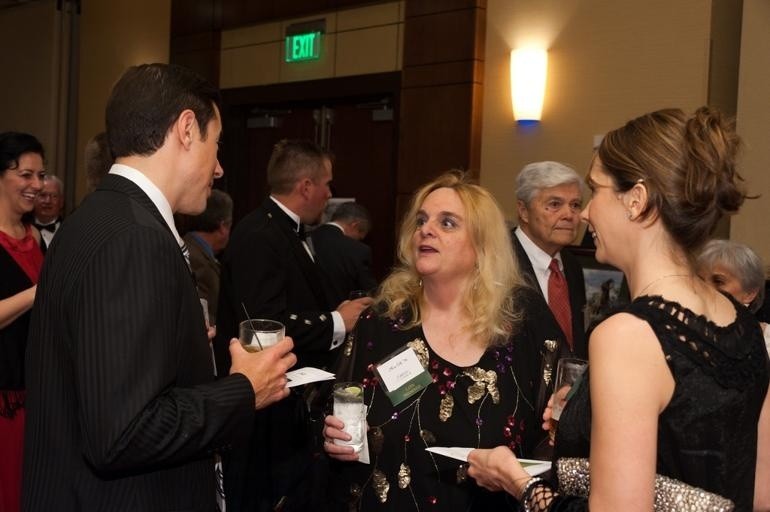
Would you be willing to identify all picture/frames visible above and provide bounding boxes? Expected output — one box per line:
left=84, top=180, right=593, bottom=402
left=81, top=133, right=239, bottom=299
left=572, top=248, right=632, bottom=334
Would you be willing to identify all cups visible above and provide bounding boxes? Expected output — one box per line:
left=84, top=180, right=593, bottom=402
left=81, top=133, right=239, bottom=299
left=332, top=383, right=365, bottom=454
left=544, top=357, right=590, bottom=444
left=238, top=318, right=287, bottom=353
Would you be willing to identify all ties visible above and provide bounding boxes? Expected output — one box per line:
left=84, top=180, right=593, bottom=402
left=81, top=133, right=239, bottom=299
left=548, top=259, right=575, bottom=353
left=178, top=236, right=226, bottom=512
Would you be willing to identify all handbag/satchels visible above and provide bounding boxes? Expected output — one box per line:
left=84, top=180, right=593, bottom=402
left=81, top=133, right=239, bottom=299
left=555, top=458, right=735, bottom=512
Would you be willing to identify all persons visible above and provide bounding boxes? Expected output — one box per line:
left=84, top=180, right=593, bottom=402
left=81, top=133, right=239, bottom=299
left=1, top=133, right=66, bottom=511
left=29, top=64, right=297, bottom=511
left=183, top=137, right=591, bottom=512
left=464, top=106, right=770, bottom=511
left=696, top=238, right=769, bottom=322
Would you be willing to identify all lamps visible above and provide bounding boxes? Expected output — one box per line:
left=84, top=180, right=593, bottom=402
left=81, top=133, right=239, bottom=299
left=510, top=46, right=548, bottom=126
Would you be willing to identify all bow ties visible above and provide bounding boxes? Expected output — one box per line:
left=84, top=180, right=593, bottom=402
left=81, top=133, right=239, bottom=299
left=298, top=222, right=307, bottom=241
left=35, top=224, right=55, bottom=232
